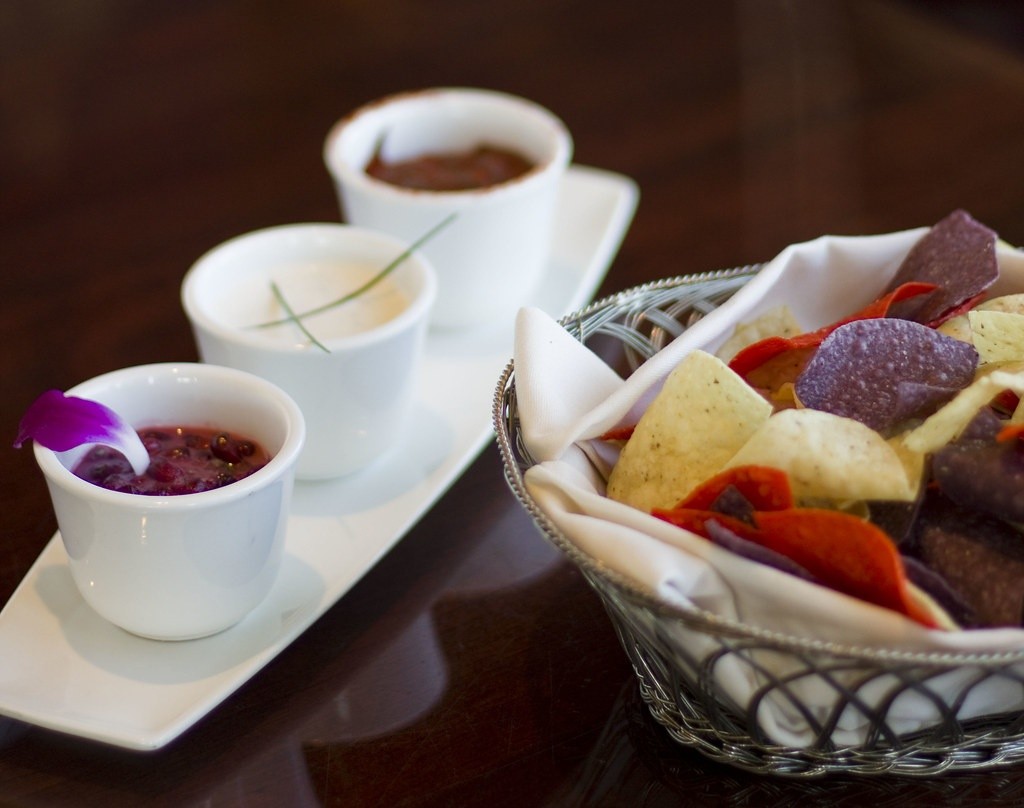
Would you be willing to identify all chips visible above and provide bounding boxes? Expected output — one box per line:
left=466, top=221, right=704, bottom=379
left=607, top=212, right=1024, bottom=642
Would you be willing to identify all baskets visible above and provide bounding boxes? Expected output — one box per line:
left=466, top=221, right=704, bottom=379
left=494, top=269, right=1023, bottom=782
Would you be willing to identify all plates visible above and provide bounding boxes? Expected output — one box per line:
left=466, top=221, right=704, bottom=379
left=0, top=168, right=640, bottom=750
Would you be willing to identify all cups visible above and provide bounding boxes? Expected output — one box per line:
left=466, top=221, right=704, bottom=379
left=33, top=364, right=305, bottom=641
left=181, top=223, right=436, bottom=481
left=324, top=89, right=571, bottom=328
